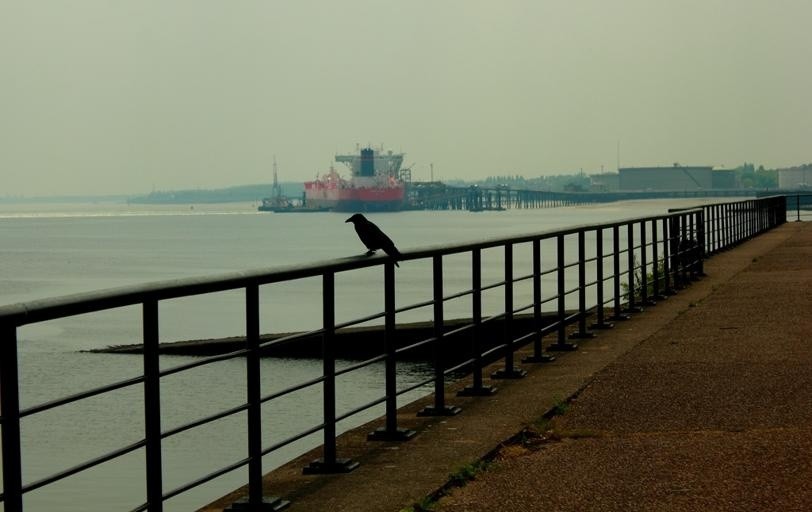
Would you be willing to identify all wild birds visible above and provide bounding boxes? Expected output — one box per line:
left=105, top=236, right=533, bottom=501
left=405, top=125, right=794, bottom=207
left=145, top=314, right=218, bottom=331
left=345, top=214, right=402, bottom=268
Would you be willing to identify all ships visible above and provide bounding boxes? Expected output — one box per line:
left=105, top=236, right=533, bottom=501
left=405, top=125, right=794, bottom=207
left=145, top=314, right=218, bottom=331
left=303, top=148, right=413, bottom=211
